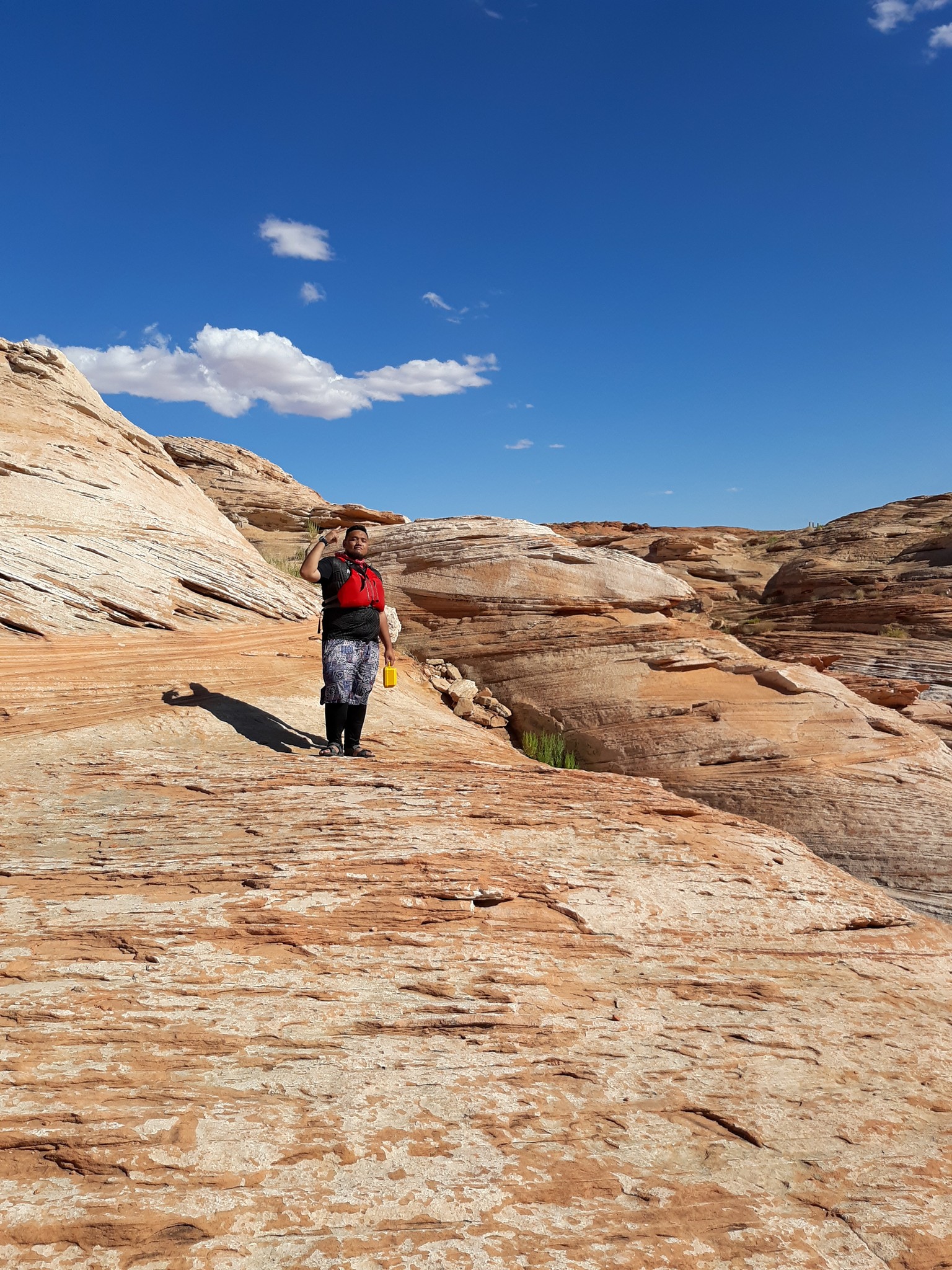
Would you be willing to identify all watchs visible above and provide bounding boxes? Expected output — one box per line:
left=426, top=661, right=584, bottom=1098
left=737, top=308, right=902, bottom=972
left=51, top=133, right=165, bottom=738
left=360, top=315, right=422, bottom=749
left=319, top=536, right=329, bottom=546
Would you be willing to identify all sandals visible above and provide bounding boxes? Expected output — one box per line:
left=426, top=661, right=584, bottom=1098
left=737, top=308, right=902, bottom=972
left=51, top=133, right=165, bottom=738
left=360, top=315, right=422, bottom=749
left=319, top=742, right=344, bottom=757
left=344, top=745, right=376, bottom=757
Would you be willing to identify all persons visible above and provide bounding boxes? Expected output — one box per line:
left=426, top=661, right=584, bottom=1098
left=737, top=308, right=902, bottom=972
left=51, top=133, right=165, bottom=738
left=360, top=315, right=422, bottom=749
left=299, top=525, right=395, bottom=758
